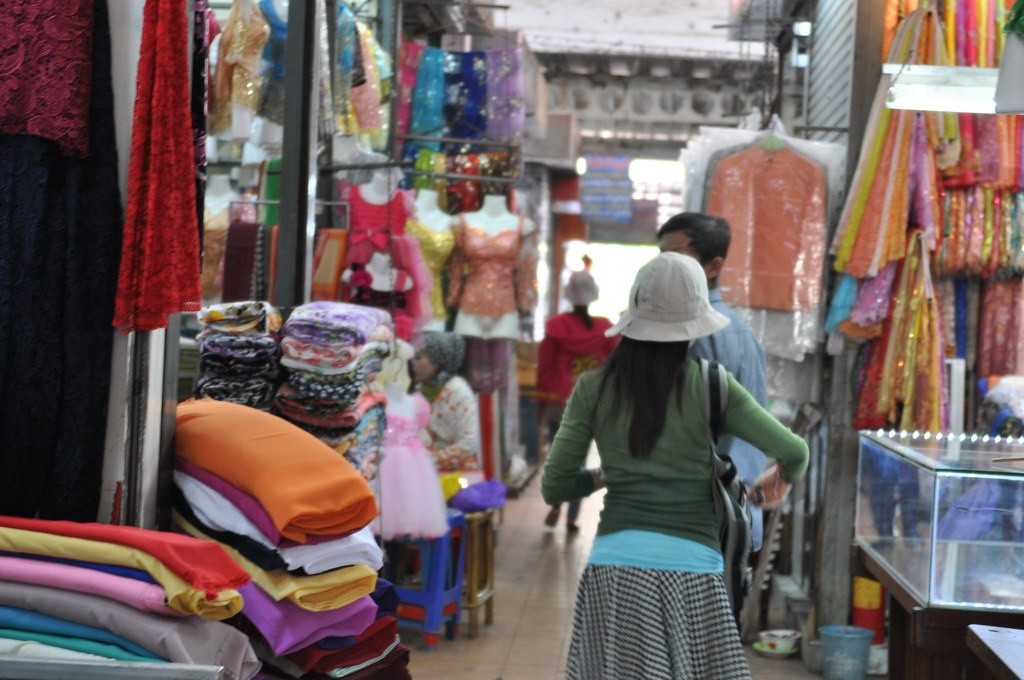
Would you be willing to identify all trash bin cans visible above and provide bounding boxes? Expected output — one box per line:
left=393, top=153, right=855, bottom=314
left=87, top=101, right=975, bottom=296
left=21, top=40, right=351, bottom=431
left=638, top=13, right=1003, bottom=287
left=817, top=626, right=876, bottom=680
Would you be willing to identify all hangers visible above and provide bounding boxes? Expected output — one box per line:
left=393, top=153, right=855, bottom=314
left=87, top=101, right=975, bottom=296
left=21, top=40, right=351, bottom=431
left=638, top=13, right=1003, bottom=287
left=751, top=115, right=790, bottom=151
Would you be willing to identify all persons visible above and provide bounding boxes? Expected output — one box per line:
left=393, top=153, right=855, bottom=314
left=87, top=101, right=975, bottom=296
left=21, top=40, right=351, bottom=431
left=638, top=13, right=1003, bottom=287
left=369, top=378, right=450, bottom=540
left=409, top=331, right=486, bottom=505
left=540, top=250, right=811, bottom=679
left=396, top=186, right=456, bottom=331
left=862, top=445, right=928, bottom=552
left=445, top=193, right=538, bottom=343
left=209, top=0, right=271, bottom=143
left=935, top=375, right=1024, bottom=576
left=251, top=1, right=289, bottom=152
left=657, top=212, right=772, bottom=640
left=538, top=270, right=622, bottom=533
left=338, top=166, right=411, bottom=312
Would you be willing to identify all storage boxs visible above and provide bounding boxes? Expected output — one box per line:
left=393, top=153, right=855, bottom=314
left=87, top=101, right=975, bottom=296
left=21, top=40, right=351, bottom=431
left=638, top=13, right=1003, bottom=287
left=854, top=429, right=1024, bottom=613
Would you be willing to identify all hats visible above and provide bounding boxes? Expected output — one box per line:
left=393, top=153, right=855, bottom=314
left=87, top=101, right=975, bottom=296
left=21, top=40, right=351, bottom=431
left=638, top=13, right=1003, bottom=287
left=604, top=251, right=731, bottom=342
left=564, top=272, right=599, bottom=306
left=414, top=330, right=466, bottom=386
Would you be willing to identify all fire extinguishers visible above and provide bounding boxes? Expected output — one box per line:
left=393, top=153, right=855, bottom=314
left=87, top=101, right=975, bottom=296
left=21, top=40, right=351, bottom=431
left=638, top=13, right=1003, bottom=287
left=853, top=570, right=887, bottom=644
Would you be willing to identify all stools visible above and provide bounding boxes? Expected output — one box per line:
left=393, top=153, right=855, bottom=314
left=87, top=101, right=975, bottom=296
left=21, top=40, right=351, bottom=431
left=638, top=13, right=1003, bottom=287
left=460, top=509, right=494, bottom=636
left=395, top=505, right=466, bottom=653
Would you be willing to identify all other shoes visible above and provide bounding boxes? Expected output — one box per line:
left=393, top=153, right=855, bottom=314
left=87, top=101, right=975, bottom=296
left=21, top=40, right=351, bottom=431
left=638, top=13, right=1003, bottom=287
left=545, top=511, right=558, bottom=526
left=568, top=525, right=577, bottom=531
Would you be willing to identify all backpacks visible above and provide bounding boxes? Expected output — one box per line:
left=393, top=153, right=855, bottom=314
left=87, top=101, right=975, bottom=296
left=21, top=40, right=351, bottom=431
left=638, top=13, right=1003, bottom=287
left=696, top=355, right=754, bottom=644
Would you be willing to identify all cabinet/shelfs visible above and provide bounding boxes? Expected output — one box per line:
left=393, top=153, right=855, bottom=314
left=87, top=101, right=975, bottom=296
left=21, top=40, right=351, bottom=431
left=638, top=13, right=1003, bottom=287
left=850, top=546, right=1024, bottom=680
